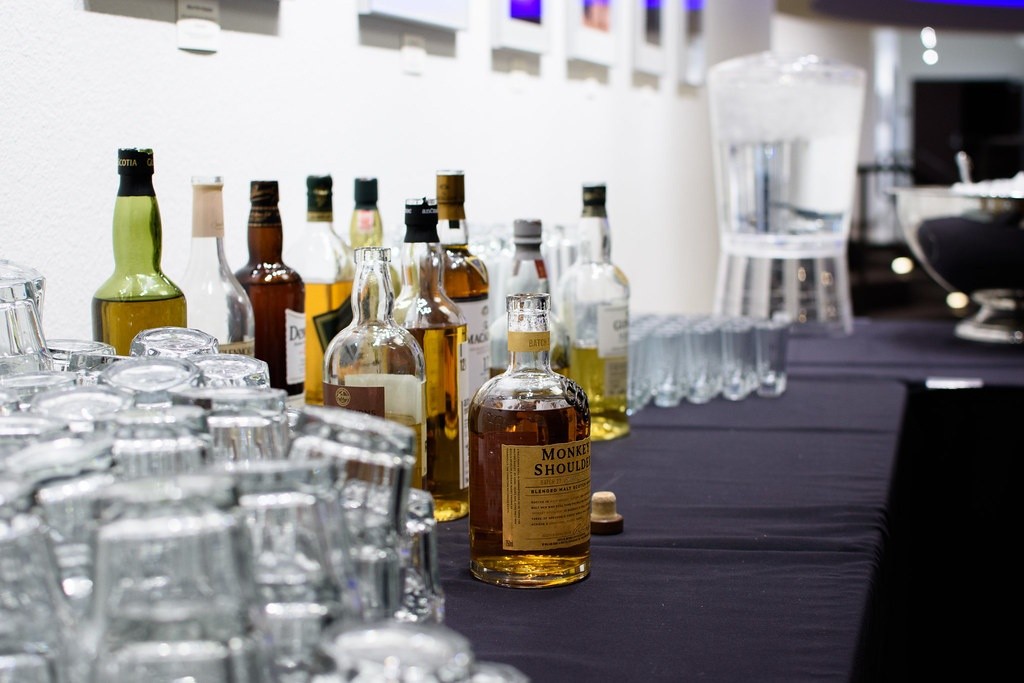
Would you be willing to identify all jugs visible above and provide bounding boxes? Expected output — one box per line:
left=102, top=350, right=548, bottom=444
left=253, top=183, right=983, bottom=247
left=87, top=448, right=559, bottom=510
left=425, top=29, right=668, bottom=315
left=711, top=55, right=866, bottom=336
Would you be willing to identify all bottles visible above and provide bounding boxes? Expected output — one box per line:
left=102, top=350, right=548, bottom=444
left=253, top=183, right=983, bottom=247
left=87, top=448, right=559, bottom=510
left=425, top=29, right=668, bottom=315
left=322, top=247, right=426, bottom=493
left=177, top=176, right=255, bottom=356
left=435, top=172, right=489, bottom=403
left=391, top=198, right=469, bottom=521
left=284, top=175, right=350, bottom=405
left=563, top=183, right=629, bottom=442
left=233, top=180, right=305, bottom=397
left=352, top=178, right=403, bottom=298
left=469, top=291, right=590, bottom=588
left=488, top=219, right=568, bottom=379
left=92, top=148, right=187, bottom=357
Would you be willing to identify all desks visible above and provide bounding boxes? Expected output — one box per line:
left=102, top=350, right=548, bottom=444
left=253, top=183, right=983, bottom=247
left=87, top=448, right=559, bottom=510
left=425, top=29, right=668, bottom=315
left=432, top=318, right=1024, bottom=683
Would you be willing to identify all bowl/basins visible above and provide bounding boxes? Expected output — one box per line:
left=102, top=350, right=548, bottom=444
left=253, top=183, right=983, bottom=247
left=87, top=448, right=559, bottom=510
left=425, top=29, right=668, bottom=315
left=881, top=185, right=1024, bottom=342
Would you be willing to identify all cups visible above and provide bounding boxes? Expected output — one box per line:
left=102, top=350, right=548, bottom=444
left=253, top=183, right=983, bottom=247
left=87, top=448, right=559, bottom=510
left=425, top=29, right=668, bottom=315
left=629, top=315, right=789, bottom=413
left=0, top=260, right=529, bottom=683
